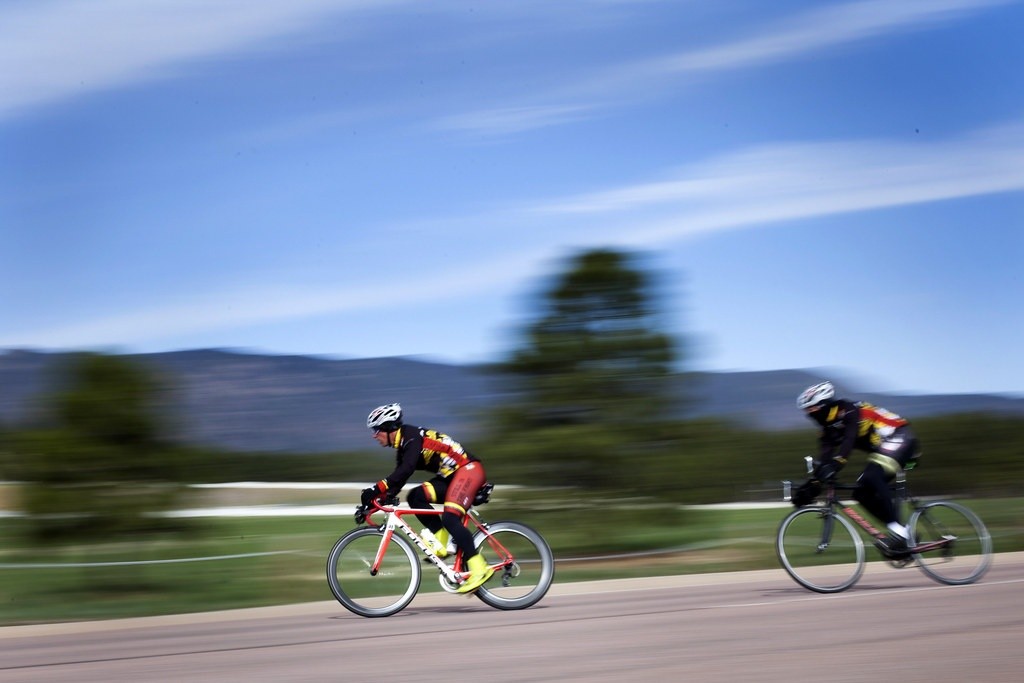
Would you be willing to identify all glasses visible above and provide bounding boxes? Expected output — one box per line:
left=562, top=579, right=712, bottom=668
left=368, top=427, right=386, bottom=435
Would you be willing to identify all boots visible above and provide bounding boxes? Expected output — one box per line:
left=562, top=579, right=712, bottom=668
left=422, top=528, right=450, bottom=558
left=454, top=547, right=496, bottom=596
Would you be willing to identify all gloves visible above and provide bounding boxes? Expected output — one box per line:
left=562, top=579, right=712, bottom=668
left=789, top=476, right=826, bottom=509
left=359, top=484, right=383, bottom=506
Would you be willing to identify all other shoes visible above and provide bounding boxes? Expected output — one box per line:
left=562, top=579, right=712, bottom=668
left=874, top=524, right=912, bottom=568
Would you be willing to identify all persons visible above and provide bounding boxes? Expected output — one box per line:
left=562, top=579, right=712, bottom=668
left=356, top=403, right=494, bottom=593
left=792, top=381, right=917, bottom=569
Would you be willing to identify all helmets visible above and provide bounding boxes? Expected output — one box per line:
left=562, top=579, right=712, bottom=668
left=366, top=403, right=403, bottom=431
left=796, top=380, right=835, bottom=410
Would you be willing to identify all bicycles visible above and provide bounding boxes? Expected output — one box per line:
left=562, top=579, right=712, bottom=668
left=776, top=463, right=992, bottom=593
left=326, top=480, right=554, bottom=617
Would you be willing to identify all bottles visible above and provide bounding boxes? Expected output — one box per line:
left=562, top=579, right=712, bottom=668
left=420, top=528, right=443, bottom=552
left=446, top=533, right=457, bottom=554
